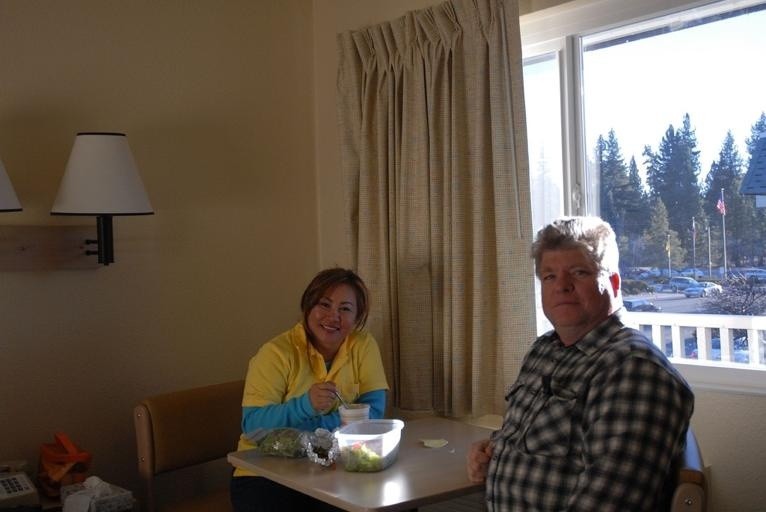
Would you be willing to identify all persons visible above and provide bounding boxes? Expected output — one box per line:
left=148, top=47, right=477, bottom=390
left=230, top=268, right=389, bottom=512
left=467, top=217, right=694, bottom=512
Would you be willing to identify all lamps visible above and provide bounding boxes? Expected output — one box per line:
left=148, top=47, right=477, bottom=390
left=0, top=132, right=154, bottom=272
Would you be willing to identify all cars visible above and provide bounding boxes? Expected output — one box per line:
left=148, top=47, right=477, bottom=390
left=614, top=262, right=765, bottom=368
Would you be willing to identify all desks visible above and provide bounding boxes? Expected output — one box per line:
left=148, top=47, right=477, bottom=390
left=227, top=418, right=498, bottom=512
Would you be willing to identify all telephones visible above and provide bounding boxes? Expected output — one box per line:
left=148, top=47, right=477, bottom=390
left=0, top=459, right=40, bottom=511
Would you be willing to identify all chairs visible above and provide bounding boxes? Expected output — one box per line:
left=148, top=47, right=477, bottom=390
left=132, top=380, right=244, bottom=511
left=665, top=426, right=710, bottom=512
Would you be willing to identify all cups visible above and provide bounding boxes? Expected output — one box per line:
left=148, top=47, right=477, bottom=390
left=339, top=403, right=371, bottom=424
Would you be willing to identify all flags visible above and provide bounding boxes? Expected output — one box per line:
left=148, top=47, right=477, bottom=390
left=665, top=238, right=670, bottom=257
left=716, top=194, right=725, bottom=214
